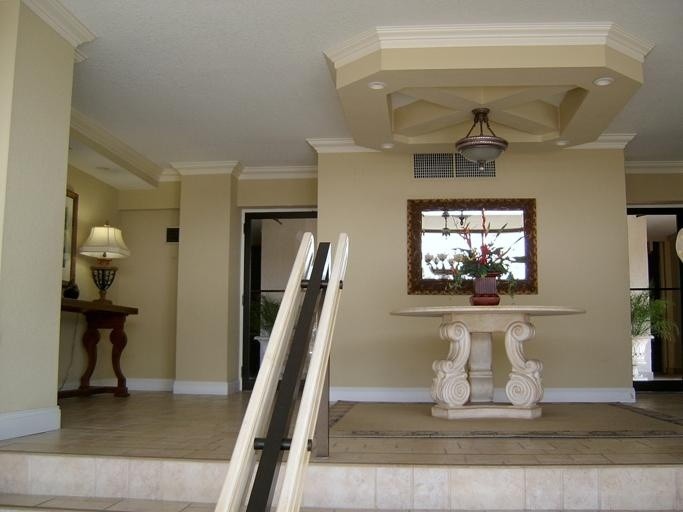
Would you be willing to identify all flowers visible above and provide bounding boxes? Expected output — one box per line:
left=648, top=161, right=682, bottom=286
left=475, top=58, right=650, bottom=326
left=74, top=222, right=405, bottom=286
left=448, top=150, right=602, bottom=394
left=446, top=209, right=528, bottom=297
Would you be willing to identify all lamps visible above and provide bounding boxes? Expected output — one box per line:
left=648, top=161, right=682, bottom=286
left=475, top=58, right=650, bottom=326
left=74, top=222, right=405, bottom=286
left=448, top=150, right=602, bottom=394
left=80, top=221, right=130, bottom=305
left=454, top=108, right=508, bottom=170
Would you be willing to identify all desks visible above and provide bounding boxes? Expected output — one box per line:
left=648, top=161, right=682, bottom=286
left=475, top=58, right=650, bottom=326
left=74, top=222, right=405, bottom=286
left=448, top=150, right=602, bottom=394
left=389, top=305, right=587, bottom=420
left=61, top=299, right=138, bottom=397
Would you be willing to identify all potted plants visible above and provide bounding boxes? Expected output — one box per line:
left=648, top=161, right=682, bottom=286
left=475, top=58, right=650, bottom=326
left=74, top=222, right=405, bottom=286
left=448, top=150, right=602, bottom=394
left=253, top=295, right=281, bottom=365
left=630, top=279, right=680, bottom=381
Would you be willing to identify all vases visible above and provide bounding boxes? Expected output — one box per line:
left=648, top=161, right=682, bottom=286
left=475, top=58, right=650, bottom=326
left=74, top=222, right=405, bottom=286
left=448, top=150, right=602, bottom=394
left=469, top=273, right=500, bottom=305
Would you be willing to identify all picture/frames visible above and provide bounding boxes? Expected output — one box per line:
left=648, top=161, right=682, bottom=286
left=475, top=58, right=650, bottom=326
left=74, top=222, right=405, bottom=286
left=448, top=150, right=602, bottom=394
left=62, top=189, right=79, bottom=289
left=406, top=199, right=538, bottom=296
